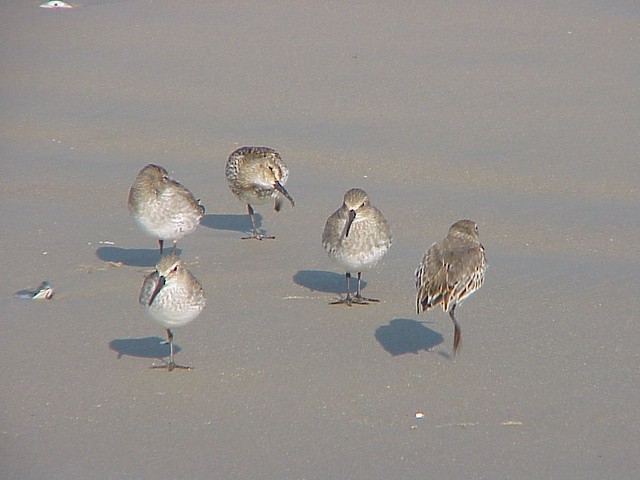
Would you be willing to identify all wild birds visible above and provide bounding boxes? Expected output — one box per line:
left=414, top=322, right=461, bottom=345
left=127, top=164, right=205, bottom=260
left=138, top=256, right=208, bottom=371
left=322, top=189, right=393, bottom=307
left=225, top=145, right=295, bottom=240
left=414, top=219, right=488, bottom=354
